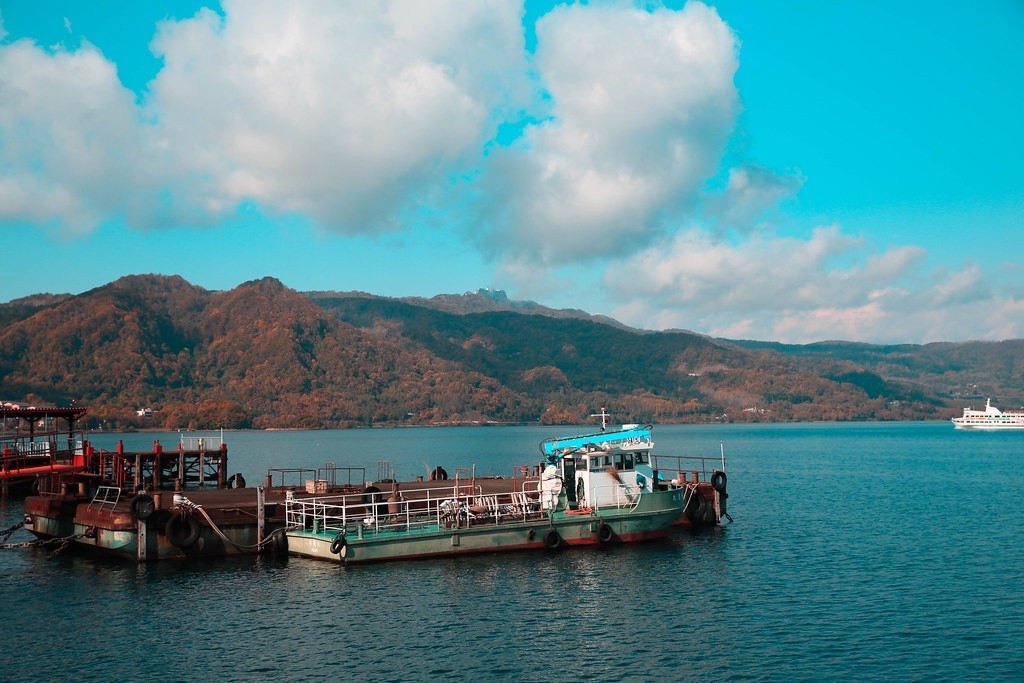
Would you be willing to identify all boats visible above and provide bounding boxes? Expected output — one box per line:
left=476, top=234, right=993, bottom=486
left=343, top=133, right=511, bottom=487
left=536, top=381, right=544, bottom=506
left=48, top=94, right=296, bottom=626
left=284, top=406, right=689, bottom=565
left=951, top=398, right=1024, bottom=430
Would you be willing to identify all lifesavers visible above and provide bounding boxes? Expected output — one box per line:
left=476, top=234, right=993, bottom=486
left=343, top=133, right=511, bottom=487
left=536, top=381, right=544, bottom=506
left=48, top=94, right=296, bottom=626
left=165, top=508, right=201, bottom=551
left=227, top=474, right=246, bottom=490
left=328, top=534, right=347, bottom=555
left=684, top=491, right=707, bottom=521
left=271, top=528, right=289, bottom=557
left=711, top=471, right=728, bottom=492
left=128, top=492, right=157, bottom=522
left=543, top=529, right=562, bottom=549
left=431, top=466, right=448, bottom=483
left=595, top=523, right=614, bottom=544
